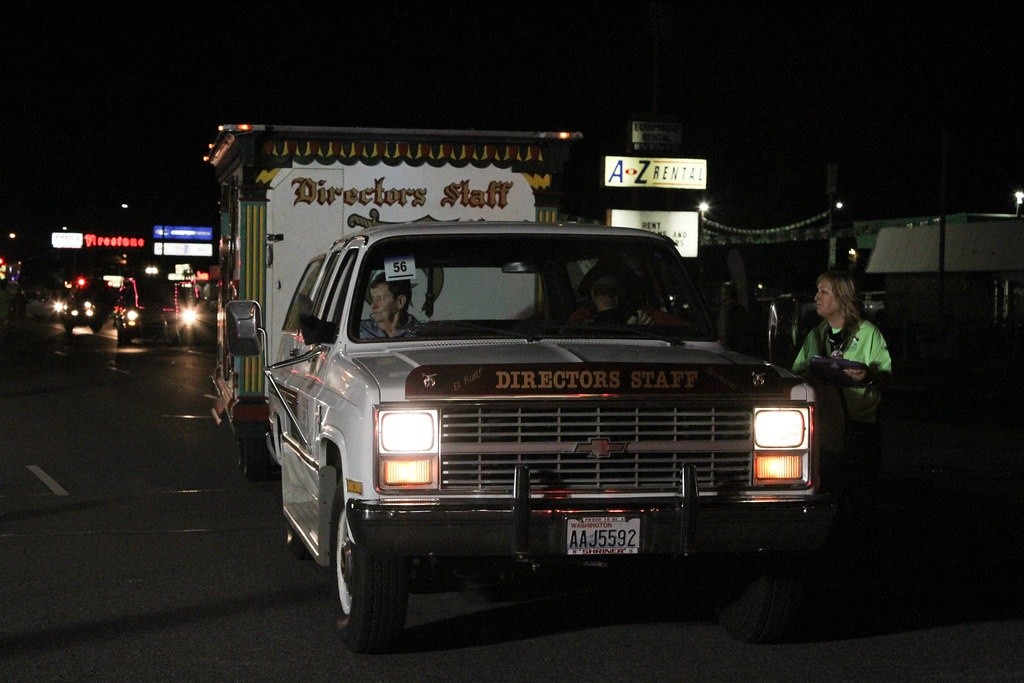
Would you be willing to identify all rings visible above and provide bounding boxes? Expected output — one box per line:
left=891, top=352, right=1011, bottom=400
left=854, top=375, right=858, bottom=379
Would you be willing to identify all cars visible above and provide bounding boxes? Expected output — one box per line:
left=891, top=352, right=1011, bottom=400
left=52, top=275, right=219, bottom=347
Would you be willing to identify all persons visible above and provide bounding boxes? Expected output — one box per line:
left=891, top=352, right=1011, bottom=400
left=792, top=272, right=894, bottom=511
left=359, top=270, right=425, bottom=338
left=567, top=262, right=657, bottom=326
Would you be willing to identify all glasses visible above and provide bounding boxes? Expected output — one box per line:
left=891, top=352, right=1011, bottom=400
left=593, top=283, right=620, bottom=299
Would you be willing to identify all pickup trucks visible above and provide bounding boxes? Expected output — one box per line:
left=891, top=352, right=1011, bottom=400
left=224, top=215, right=841, bottom=654
